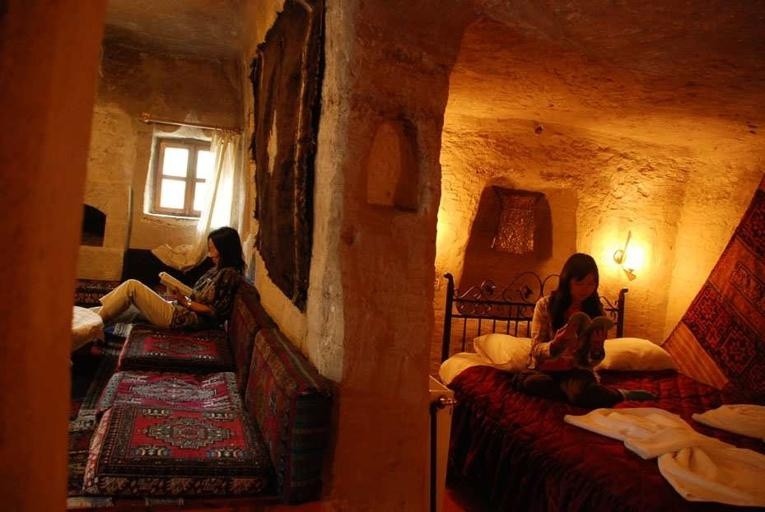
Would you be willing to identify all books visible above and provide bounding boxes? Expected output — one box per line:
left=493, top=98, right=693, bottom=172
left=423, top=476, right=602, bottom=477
left=566, top=311, right=613, bottom=338
left=158, top=271, right=193, bottom=297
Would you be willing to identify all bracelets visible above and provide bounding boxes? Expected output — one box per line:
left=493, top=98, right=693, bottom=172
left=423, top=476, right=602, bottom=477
left=186, top=300, right=192, bottom=308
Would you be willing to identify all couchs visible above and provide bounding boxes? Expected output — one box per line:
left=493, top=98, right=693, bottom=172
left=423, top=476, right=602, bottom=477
left=81, top=273, right=332, bottom=511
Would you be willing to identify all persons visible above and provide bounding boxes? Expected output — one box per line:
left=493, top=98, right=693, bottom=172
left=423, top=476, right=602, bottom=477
left=89, top=227, right=245, bottom=330
left=524, top=253, right=658, bottom=407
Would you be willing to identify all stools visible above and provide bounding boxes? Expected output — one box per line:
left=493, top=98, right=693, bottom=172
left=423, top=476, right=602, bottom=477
left=70, top=304, right=105, bottom=399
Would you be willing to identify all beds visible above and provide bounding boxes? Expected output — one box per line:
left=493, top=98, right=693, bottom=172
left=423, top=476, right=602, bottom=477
left=438, top=270, right=765, bottom=509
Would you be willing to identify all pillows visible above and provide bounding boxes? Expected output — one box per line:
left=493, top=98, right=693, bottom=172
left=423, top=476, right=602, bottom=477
left=473, top=331, right=682, bottom=375
